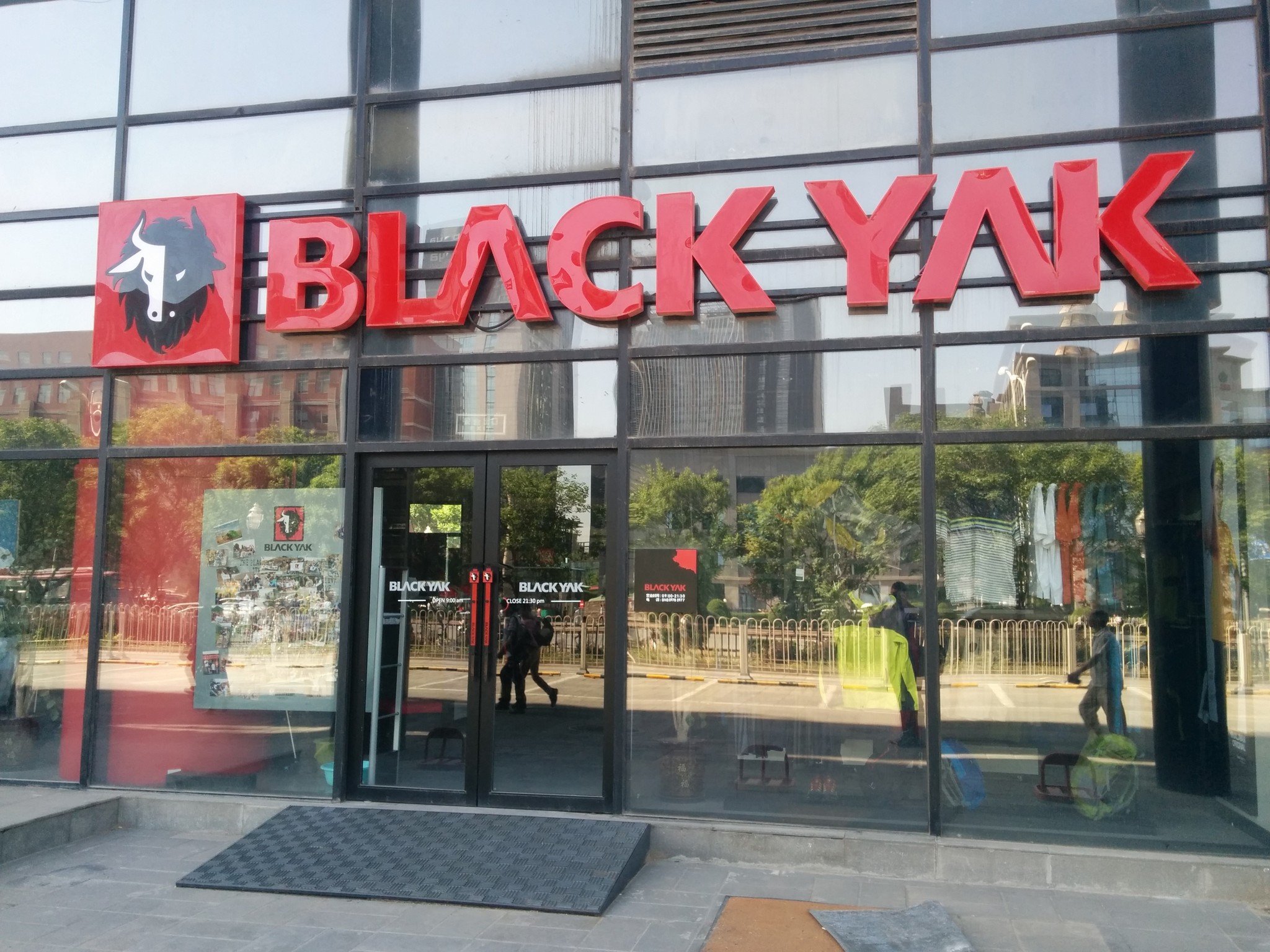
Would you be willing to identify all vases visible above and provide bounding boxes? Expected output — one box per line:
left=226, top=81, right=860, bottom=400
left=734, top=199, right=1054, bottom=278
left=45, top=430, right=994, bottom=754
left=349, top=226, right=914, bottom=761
left=659, top=737, right=709, bottom=798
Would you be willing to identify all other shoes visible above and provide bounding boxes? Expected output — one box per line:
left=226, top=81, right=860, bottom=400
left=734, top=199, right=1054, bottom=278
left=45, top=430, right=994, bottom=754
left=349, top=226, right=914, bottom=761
left=511, top=706, right=527, bottom=713
left=496, top=703, right=509, bottom=712
left=549, top=688, right=558, bottom=706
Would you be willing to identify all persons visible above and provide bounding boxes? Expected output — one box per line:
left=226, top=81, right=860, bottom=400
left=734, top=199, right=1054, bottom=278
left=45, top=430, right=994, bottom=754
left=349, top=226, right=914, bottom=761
left=866, top=581, right=923, bottom=749
left=1068, top=611, right=1126, bottom=738
left=1201, top=457, right=1241, bottom=725
left=495, top=597, right=559, bottom=716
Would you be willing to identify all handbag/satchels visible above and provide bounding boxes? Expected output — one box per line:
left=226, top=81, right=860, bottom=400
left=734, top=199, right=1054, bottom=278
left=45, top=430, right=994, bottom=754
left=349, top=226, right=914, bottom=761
left=506, top=616, right=538, bottom=658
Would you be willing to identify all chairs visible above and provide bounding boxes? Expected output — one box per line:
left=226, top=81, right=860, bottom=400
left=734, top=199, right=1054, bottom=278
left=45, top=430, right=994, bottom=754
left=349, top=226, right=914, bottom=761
left=417, top=726, right=466, bottom=758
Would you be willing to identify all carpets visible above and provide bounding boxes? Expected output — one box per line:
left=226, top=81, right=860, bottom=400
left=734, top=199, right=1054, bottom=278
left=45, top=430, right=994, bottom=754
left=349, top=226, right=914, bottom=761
left=699, top=895, right=979, bottom=952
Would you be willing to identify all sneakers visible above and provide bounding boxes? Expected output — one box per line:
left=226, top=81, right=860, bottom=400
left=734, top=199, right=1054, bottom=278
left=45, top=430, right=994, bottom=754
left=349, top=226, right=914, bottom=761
left=806, top=775, right=823, bottom=802
left=821, top=776, right=838, bottom=803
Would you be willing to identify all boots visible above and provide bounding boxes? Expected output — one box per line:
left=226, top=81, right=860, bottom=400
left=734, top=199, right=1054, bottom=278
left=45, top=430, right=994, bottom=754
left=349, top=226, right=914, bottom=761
left=891, top=709, right=920, bottom=747
